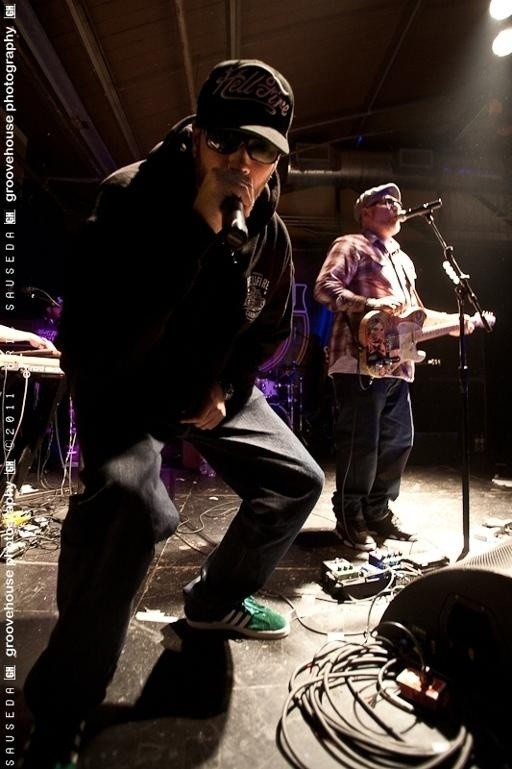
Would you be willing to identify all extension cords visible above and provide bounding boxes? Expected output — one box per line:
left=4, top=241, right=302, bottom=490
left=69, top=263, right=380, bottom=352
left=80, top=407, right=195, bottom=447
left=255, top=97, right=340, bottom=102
left=395, top=666, right=448, bottom=709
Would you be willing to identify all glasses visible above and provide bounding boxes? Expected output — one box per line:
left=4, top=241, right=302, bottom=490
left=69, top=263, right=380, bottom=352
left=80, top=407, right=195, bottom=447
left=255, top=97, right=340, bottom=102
left=201, top=127, right=286, bottom=165
left=379, top=197, right=403, bottom=210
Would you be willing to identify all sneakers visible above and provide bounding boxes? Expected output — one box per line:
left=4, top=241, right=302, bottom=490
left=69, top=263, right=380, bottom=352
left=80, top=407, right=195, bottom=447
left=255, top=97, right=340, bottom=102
left=366, top=509, right=418, bottom=542
left=185, top=594, right=290, bottom=640
left=14, top=704, right=89, bottom=766
left=334, top=511, right=377, bottom=552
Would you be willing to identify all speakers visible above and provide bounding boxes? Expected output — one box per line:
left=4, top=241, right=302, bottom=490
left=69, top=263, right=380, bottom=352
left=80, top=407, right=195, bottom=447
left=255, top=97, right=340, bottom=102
left=374, top=537, right=512, bottom=694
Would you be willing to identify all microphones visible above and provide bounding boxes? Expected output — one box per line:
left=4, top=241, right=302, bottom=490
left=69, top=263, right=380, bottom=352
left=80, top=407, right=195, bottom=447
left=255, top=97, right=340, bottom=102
left=220, top=196, right=248, bottom=249
left=396, top=199, right=443, bottom=223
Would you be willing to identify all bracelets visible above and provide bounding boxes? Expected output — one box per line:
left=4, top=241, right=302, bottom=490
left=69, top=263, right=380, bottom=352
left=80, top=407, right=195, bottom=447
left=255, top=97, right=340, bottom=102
left=365, top=297, right=376, bottom=312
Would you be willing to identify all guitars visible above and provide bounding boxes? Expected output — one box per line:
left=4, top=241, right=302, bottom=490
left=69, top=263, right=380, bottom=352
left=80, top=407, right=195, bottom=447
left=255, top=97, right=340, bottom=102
left=358, top=304, right=496, bottom=380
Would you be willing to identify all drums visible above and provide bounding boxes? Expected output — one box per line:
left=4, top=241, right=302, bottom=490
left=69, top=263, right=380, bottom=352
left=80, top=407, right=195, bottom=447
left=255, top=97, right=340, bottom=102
left=266, top=400, right=293, bottom=432
left=254, top=373, right=281, bottom=402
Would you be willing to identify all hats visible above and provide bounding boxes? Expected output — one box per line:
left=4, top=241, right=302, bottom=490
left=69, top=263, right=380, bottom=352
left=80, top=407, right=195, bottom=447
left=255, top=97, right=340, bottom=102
left=196, top=59, right=296, bottom=157
left=353, top=182, right=401, bottom=226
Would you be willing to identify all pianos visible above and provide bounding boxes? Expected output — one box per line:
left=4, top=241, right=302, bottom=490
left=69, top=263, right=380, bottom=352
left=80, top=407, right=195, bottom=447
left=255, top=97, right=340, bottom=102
left=0, top=353, right=65, bottom=380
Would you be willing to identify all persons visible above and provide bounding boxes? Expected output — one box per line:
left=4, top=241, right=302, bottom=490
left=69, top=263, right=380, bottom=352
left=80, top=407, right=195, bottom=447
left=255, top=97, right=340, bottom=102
left=27, top=296, right=68, bottom=473
left=22, top=59, right=324, bottom=768
left=313, top=183, right=496, bottom=552
